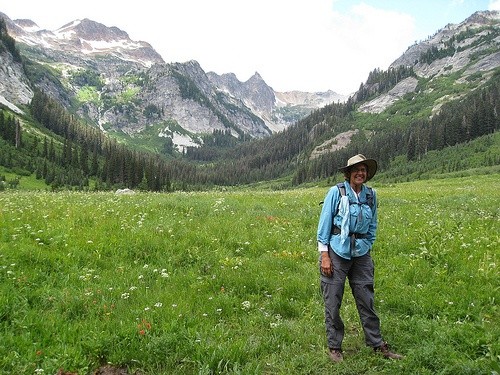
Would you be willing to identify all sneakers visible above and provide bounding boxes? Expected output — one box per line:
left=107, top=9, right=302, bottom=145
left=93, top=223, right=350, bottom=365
left=330, top=349, right=344, bottom=363
left=373, top=344, right=403, bottom=360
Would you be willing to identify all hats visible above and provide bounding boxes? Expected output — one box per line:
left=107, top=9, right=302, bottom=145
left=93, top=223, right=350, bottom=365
left=339, top=153, right=378, bottom=183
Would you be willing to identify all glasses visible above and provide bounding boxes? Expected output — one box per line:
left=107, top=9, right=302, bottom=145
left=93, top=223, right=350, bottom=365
left=351, top=168, right=368, bottom=172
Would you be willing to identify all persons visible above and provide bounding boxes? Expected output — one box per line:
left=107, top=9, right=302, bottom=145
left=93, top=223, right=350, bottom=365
left=316, top=154, right=403, bottom=364
